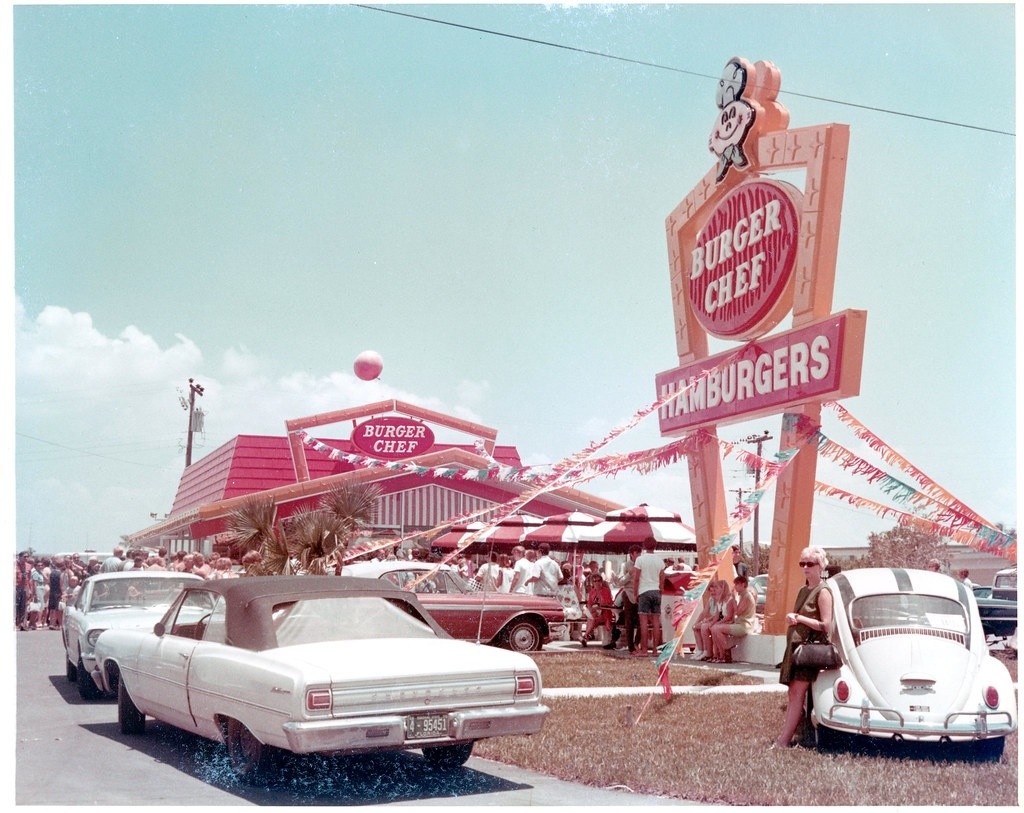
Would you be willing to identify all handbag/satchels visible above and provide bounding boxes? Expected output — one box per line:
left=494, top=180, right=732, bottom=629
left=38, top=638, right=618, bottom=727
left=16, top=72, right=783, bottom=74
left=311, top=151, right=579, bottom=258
left=30, top=597, right=40, bottom=612
left=586, top=604, right=602, bottom=619
left=58, top=598, right=66, bottom=611
left=790, top=621, right=843, bottom=669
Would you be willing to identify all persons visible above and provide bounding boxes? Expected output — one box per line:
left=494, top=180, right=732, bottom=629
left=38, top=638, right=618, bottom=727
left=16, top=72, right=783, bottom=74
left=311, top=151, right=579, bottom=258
left=689, top=576, right=756, bottom=664
left=617, top=537, right=699, bottom=657
left=958, top=569, right=974, bottom=589
left=928, top=558, right=941, bottom=572
left=580, top=575, right=614, bottom=647
left=358, top=542, right=616, bottom=621
left=16, top=545, right=263, bottom=632
left=769, top=546, right=834, bottom=750
left=731, top=545, right=749, bottom=578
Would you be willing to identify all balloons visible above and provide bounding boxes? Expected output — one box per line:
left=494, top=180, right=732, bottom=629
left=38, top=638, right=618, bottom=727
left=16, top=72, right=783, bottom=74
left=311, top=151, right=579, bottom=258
left=353, top=350, right=383, bottom=382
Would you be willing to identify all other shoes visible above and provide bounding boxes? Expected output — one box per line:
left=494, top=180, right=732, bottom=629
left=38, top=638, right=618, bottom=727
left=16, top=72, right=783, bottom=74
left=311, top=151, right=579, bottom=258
left=581, top=640, right=587, bottom=647
left=603, top=642, right=617, bottom=650
left=49, top=625, right=56, bottom=629
left=20, top=627, right=27, bottom=631
left=690, top=650, right=725, bottom=663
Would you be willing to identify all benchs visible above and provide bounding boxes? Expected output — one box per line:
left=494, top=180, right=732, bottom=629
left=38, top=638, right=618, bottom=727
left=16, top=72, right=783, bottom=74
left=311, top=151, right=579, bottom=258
left=549, top=622, right=603, bottom=641
left=730, top=634, right=787, bottom=668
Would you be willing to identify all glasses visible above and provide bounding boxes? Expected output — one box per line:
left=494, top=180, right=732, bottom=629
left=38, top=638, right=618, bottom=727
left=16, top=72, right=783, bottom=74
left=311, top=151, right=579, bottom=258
left=799, top=561, right=819, bottom=568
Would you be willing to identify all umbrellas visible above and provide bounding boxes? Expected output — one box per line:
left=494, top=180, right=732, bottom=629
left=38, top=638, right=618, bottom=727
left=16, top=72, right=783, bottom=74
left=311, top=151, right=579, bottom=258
left=581, top=503, right=696, bottom=544
left=431, top=519, right=488, bottom=548
left=475, top=510, right=543, bottom=544
left=519, top=509, right=606, bottom=585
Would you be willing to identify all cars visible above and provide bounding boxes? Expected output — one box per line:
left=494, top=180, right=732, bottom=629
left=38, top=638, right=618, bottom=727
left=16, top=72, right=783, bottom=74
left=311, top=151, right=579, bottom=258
left=972, top=586, right=992, bottom=599
left=808, top=568, right=1017, bottom=763
left=976, top=598, right=1017, bottom=636
left=750, top=574, right=768, bottom=613
left=325, top=559, right=565, bottom=656
left=748, top=576, right=753, bottom=583
left=58, top=571, right=227, bottom=701
left=992, top=568, right=1017, bottom=601
left=94, top=574, right=549, bottom=788
